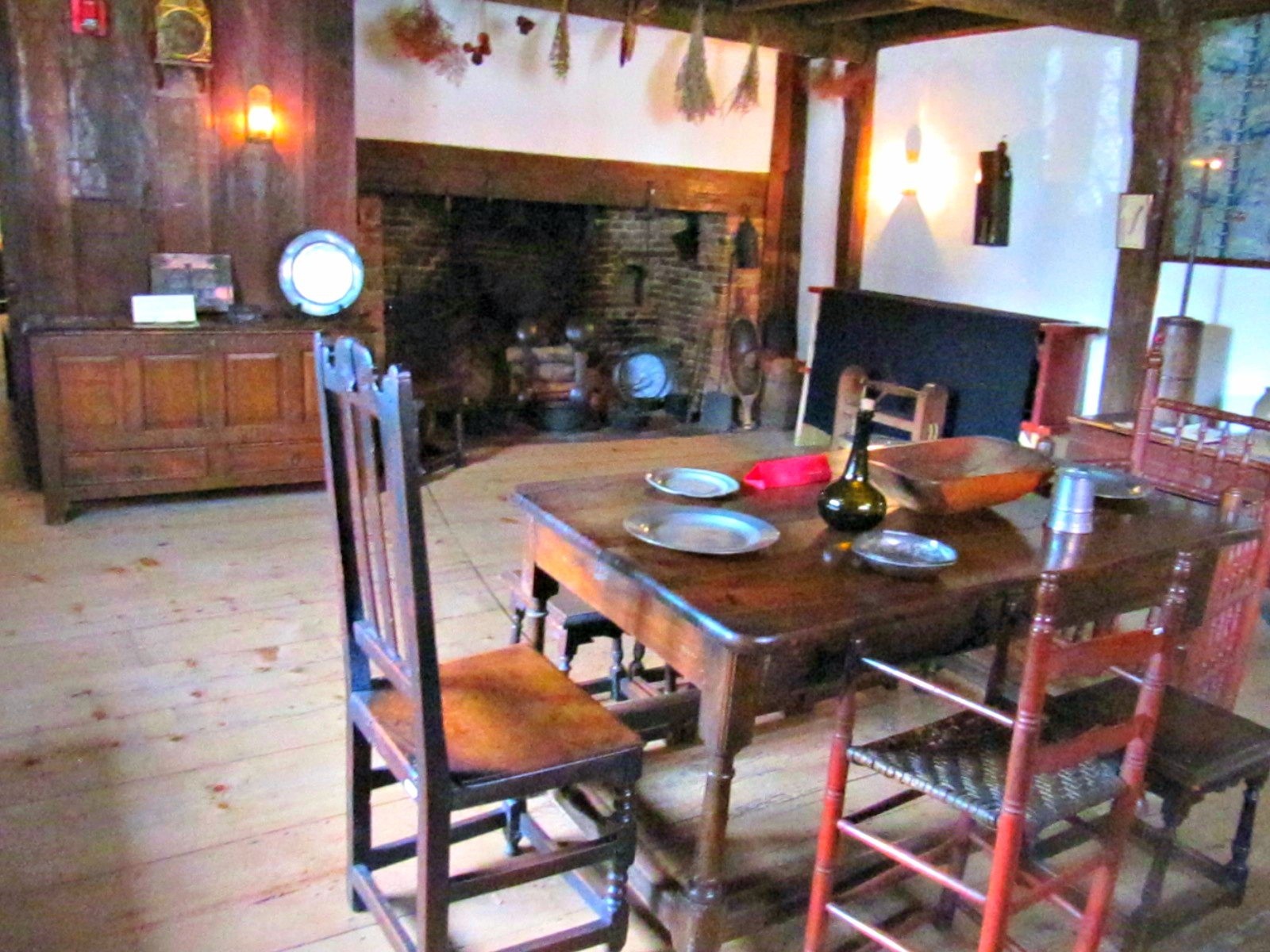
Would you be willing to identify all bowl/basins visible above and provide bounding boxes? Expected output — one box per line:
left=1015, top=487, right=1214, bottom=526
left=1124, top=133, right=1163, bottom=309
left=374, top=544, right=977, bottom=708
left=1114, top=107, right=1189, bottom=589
left=866, top=435, right=1055, bottom=514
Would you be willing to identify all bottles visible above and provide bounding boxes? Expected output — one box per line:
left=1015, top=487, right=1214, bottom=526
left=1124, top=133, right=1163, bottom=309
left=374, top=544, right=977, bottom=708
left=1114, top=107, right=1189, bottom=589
left=817, top=398, right=887, bottom=531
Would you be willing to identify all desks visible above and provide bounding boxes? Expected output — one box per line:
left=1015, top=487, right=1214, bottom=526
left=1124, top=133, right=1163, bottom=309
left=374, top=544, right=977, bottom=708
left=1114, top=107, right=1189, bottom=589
left=509, top=435, right=1269, bottom=952
left=31, top=327, right=324, bottom=524
left=1064, top=410, right=1270, bottom=711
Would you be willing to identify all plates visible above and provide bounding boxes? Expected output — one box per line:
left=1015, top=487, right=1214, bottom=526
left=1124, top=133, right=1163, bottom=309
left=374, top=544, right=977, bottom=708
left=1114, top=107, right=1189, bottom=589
left=1048, top=464, right=1154, bottom=499
left=851, top=529, right=957, bottom=581
left=623, top=505, right=780, bottom=554
left=644, top=467, right=740, bottom=498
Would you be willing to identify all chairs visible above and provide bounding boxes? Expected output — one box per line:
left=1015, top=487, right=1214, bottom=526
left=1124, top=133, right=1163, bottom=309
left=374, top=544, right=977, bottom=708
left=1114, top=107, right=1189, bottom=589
left=805, top=549, right=1192, bottom=952
left=311, top=332, right=640, bottom=952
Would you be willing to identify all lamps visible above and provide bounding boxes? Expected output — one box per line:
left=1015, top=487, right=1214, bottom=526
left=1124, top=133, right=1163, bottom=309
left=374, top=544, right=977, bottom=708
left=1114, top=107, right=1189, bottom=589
left=244, top=82, right=274, bottom=147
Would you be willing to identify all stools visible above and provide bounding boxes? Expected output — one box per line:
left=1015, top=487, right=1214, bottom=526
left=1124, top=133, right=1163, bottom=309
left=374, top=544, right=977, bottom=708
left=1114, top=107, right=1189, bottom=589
left=1023, top=674, right=1270, bottom=951
left=500, top=570, right=685, bottom=747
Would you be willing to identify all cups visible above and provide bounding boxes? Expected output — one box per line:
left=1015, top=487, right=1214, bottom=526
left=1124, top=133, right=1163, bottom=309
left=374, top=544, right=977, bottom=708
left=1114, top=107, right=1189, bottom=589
left=1044, top=469, right=1095, bottom=534
